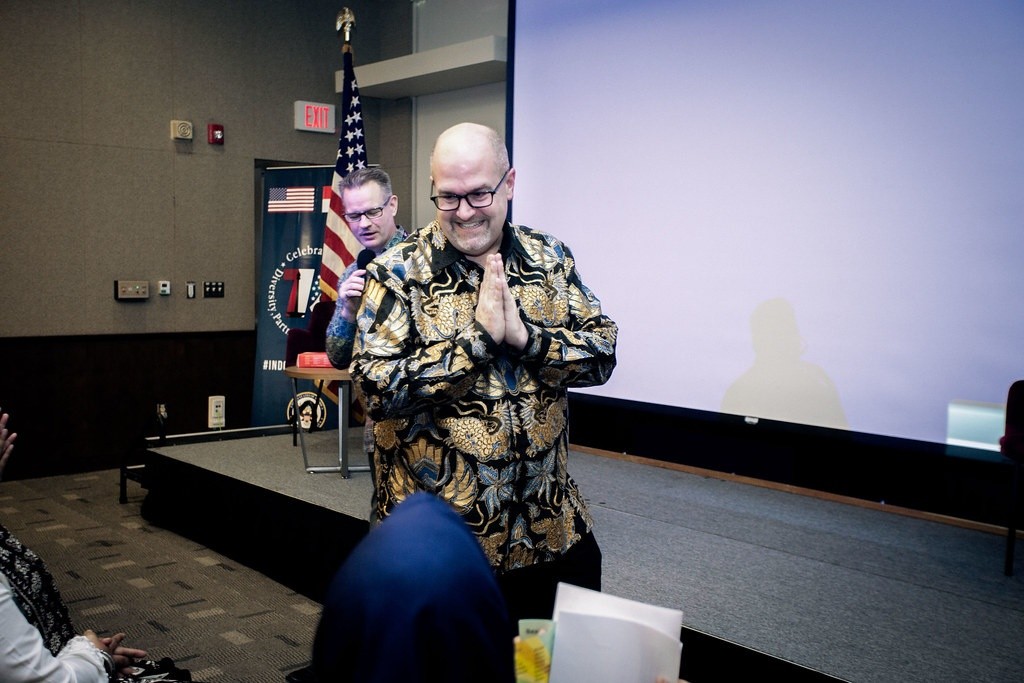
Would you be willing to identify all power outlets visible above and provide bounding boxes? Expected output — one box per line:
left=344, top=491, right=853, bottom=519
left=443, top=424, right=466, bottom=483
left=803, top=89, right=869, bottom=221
left=208, top=395, right=226, bottom=428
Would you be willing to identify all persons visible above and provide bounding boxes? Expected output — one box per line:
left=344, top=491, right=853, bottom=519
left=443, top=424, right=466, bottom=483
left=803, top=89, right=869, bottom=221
left=327, top=170, right=407, bottom=534
left=306, top=490, right=693, bottom=683
left=0, top=414, right=194, bottom=683
left=350, top=121, right=619, bottom=620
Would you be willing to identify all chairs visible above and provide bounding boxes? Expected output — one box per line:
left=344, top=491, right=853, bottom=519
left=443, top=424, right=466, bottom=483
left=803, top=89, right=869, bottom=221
left=285, top=301, right=336, bottom=446
left=1001, top=380, right=1024, bottom=578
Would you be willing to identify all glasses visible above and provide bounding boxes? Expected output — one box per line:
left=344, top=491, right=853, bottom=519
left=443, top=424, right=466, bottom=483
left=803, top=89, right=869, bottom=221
left=430, top=168, right=510, bottom=212
left=341, top=196, right=392, bottom=223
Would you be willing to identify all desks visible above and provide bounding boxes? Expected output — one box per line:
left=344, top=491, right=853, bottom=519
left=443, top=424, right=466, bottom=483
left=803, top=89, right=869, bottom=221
left=284, top=366, right=370, bottom=479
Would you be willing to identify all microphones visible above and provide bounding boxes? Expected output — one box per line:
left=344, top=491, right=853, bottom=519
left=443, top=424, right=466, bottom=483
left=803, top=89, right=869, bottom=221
left=357, top=249, right=376, bottom=279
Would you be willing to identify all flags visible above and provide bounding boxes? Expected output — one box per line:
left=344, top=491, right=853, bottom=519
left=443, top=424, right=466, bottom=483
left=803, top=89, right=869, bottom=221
left=320, top=43, right=366, bottom=304
